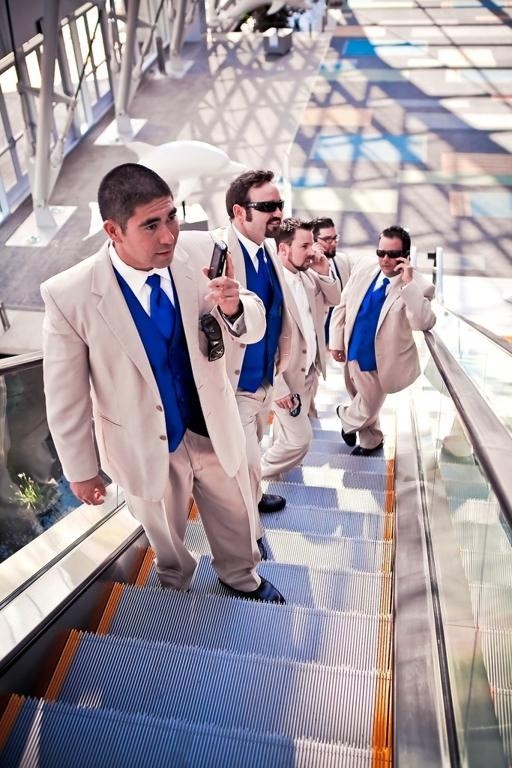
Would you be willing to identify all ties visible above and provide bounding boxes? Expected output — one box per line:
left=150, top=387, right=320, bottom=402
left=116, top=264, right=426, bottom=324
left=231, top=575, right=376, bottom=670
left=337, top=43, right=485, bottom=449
left=145, top=274, right=176, bottom=344
left=255, top=248, right=273, bottom=305
left=371, top=278, right=390, bottom=312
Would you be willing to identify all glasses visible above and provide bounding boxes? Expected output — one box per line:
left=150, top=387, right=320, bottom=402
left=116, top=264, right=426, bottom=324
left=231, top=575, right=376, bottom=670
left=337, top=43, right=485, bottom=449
left=289, top=394, right=301, bottom=417
left=316, top=236, right=338, bottom=244
left=376, top=250, right=402, bottom=258
left=237, top=202, right=283, bottom=213
left=201, top=313, right=224, bottom=362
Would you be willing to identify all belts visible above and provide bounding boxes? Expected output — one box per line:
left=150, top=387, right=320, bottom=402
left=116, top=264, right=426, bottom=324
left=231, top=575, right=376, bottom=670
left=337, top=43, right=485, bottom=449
left=306, top=362, right=314, bottom=379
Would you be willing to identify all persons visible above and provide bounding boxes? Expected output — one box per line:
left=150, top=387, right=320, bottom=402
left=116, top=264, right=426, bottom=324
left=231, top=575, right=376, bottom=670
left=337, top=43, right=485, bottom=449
left=263, top=218, right=343, bottom=479
left=36, top=163, right=290, bottom=607
left=0, top=366, right=68, bottom=560
left=329, top=223, right=437, bottom=457
left=306, top=219, right=352, bottom=417
left=186, top=167, right=294, bottom=564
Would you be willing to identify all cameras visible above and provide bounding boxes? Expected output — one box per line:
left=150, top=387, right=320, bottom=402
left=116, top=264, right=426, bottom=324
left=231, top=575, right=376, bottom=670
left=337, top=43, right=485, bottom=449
left=397, top=249, right=410, bottom=270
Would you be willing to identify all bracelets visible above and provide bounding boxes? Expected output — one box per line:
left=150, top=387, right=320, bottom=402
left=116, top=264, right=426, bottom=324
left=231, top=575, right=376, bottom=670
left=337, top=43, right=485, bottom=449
left=216, top=301, right=243, bottom=320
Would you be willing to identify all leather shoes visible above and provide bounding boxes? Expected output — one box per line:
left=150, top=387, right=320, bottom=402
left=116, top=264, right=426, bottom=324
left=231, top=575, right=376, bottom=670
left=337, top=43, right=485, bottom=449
left=337, top=405, right=356, bottom=447
left=256, top=538, right=267, bottom=561
left=351, top=439, right=383, bottom=457
left=259, top=493, right=286, bottom=513
left=218, top=576, right=285, bottom=604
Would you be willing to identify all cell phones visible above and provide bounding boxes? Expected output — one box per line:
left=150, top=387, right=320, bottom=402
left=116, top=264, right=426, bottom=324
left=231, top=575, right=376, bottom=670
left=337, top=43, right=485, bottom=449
left=208, top=241, right=227, bottom=279
